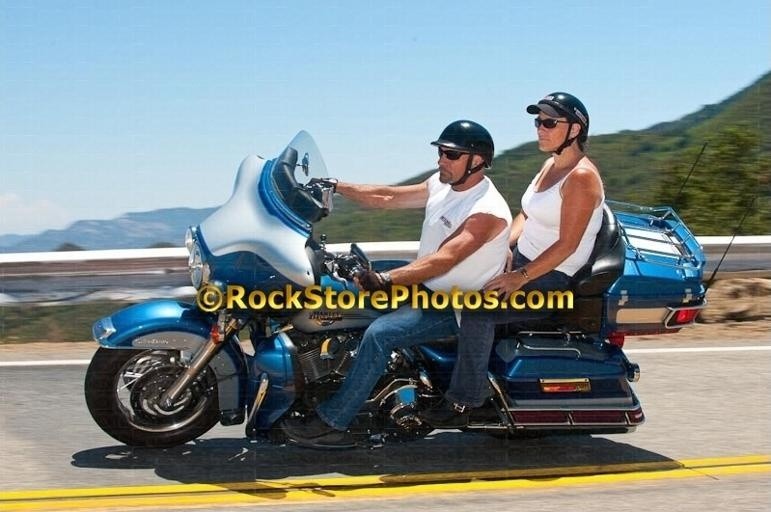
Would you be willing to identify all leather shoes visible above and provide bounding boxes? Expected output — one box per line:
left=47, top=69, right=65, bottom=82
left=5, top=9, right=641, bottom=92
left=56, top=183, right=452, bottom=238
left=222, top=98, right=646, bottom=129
left=280, top=413, right=345, bottom=445
left=419, top=397, right=497, bottom=426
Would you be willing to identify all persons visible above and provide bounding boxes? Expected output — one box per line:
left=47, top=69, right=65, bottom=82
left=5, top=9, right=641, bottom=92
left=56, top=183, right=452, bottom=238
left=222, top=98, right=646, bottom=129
left=415, top=92, right=605, bottom=429
left=281, top=120, right=514, bottom=444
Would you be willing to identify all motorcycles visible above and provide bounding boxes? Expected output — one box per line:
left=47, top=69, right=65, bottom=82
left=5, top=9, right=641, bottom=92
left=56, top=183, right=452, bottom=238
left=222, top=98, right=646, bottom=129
left=83, top=112, right=762, bottom=452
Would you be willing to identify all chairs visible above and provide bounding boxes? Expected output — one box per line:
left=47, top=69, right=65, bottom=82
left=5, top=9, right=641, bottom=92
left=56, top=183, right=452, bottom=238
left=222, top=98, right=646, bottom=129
left=512, top=203, right=627, bottom=336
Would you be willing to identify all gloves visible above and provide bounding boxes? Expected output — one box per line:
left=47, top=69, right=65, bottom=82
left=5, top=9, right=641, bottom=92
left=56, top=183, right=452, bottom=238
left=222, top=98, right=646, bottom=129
left=354, top=270, right=393, bottom=291
left=306, top=178, right=338, bottom=193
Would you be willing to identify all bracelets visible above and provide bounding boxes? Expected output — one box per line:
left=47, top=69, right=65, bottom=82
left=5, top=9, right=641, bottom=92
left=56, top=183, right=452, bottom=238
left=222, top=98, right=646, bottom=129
left=379, top=269, right=392, bottom=289
left=520, top=266, right=531, bottom=281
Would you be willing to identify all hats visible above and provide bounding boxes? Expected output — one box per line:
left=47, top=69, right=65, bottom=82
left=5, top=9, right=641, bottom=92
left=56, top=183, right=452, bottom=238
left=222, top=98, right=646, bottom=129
left=527, top=103, right=565, bottom=117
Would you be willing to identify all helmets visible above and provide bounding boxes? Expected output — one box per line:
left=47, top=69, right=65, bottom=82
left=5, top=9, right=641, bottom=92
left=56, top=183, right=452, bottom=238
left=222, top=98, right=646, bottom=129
left=430, top=120, right=494, bottom=169
left=539, top=93, right=589, bottom=136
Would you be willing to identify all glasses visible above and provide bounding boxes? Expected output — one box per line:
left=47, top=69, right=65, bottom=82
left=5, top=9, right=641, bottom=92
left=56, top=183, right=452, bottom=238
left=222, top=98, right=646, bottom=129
left=535, top=116, right=569, bottom=128
left=438, top=147, right=478, bottom=160
